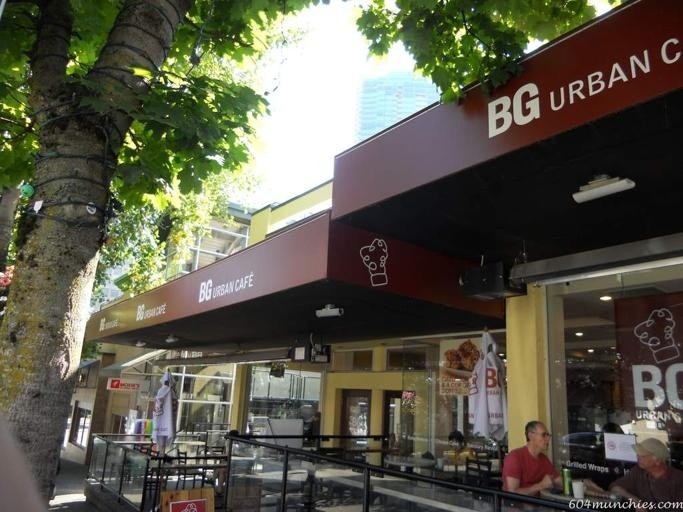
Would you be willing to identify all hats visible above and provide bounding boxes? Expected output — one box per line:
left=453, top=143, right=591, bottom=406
left=630, top=438, right=671, bottom=460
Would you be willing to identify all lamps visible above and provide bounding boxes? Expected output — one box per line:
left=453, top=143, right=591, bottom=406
left=315, top=304, right=346, bottom=318
left=572, top=174, right=642, bottom=204
left=135, top=334, right=178, bottom=347
left=292, top=343, right=332, bottom=365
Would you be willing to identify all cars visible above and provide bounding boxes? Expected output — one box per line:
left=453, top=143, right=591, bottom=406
left=560, top=432, right=638, bottom=475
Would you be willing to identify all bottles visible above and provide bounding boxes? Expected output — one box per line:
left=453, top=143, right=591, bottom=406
left=561, top=468, right=572, bottom=497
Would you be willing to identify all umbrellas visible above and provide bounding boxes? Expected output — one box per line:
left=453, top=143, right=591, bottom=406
left=150, top=366, right=178, bottom=457
left=466, top=328, right=507, bottom=457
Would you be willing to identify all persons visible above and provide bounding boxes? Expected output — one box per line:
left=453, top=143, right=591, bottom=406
left=213, top=429, right=239, bottom=496
left=607, top=437, right=682, bottom=512
left=500, top=419, right=565, bottom=512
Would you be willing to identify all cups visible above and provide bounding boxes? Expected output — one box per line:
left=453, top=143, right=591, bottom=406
left=572, top=482, right=585, bottom=499
left=155, top=434, right=167, bottom=457
